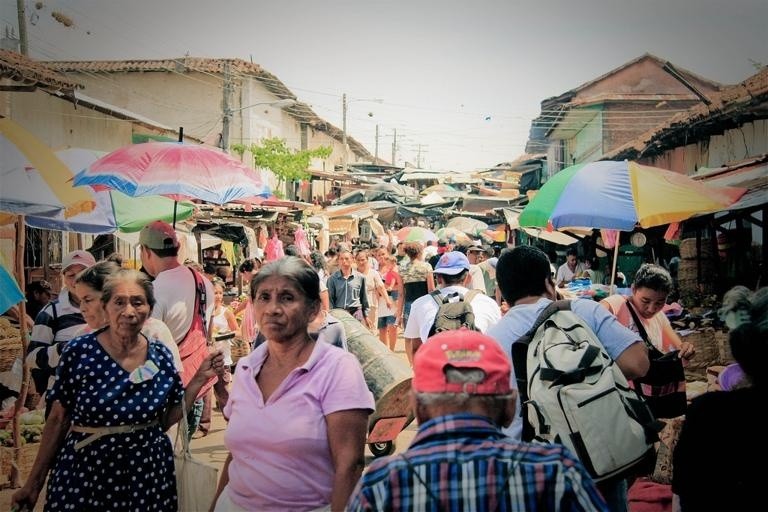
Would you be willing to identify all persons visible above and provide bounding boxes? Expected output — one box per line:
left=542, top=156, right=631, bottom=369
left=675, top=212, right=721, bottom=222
left=9, top=270, right=225, bottom=511
left=211, top=255, right=376, bottom=512
left=670, top=324, right=766, bottom=511
left=24, top=176, right=768, bottom=439
left=341, top=327, right=611, bottom=511
left=486, top=244, right=653, bottom=512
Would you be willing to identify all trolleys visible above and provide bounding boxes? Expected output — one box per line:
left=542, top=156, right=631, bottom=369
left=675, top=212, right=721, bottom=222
left=366, top=417, right=408, bottom=456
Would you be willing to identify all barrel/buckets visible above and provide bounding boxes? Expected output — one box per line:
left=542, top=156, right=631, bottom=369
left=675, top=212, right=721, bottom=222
left=630, top=233, right=646, bottom=247
left=328, top=306, right=418, bottom=441
left=717, top=364, right=744, bottom=390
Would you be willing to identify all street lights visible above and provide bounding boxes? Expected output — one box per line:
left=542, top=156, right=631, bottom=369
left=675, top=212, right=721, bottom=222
left=342, top=95, right=384, bottom=164
left=220, top=61, right=296, bottom=152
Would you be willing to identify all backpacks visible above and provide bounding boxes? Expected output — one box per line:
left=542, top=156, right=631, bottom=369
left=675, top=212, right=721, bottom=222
left=428, top=289, right=486, bottom=338
left=512, top=300, right=661, bottom=489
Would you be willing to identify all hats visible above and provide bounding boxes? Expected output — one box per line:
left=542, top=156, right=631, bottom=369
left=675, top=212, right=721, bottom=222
left=411, top=326, right=512, bottom=395
left=133, top=220, right=178, bottom=249
left=430, top=251, right=470, bottom=275
left=61, top=250, right=96, bottom=272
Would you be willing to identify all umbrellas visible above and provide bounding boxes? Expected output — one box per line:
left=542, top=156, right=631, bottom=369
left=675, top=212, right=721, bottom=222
left=21, top=146, right=195, bottom=234
left=62, top=125, right=277, bottom=232
left=521, top=158, right=751, bottom=295
left=0, top=116, right=97, bottom=221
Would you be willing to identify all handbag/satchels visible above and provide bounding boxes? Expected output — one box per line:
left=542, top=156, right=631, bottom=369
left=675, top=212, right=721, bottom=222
left=623, top=350, right=688, bottom=418
left=174, top=454, right=218, bottom=512
left=177, top=265, right=219, bottom=400
